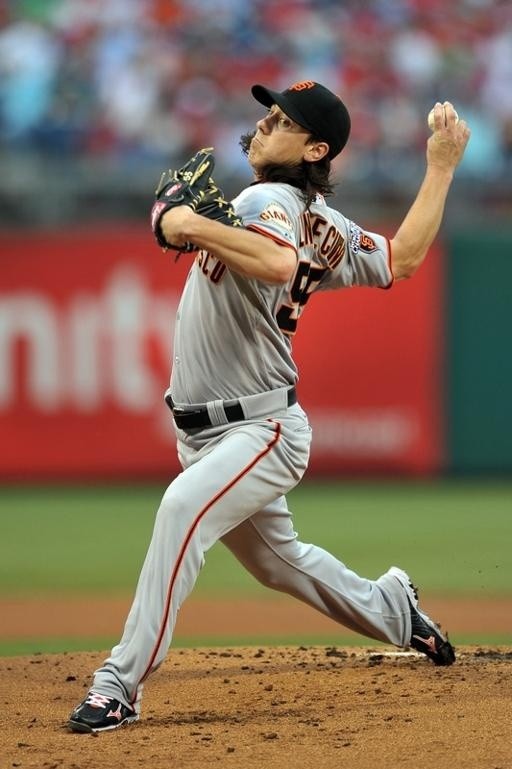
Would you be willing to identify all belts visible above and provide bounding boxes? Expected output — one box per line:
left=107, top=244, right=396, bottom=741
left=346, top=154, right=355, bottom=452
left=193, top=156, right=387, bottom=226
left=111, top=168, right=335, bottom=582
left=165, top=386, right=298, bottom=430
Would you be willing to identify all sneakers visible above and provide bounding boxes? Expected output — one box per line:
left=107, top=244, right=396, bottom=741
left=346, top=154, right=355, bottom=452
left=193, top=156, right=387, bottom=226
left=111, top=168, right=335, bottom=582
left=68, top=692, right=139, bottom=732
left=386, top=566, right=455, bottom=667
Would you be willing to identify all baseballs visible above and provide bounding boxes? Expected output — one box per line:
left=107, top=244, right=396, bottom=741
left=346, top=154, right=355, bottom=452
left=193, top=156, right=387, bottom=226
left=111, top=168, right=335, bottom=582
left=427, top=106, right=459, bottom=132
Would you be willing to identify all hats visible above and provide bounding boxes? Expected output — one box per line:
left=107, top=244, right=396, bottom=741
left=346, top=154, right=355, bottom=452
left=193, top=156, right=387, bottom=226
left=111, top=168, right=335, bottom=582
left=249, top=82, right=352, bottom=160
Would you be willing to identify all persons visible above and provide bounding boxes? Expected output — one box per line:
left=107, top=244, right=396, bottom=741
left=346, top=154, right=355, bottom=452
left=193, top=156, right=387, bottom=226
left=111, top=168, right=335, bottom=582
left=63, top=79, right=472, bottom=735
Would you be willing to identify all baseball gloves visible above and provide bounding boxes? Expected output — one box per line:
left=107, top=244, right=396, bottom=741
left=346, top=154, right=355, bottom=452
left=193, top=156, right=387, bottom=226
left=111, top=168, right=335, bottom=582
left=150, top=149, right=246, bottom=253
